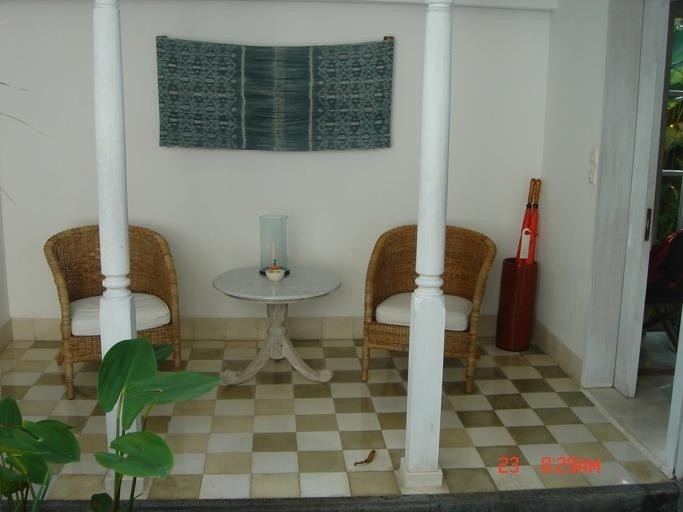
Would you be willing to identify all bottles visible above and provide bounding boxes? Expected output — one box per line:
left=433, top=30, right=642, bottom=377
left=260, top=214, right=289, bottom=277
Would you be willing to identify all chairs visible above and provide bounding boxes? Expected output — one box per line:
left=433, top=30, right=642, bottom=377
left=360, top=222, right=496, bottom=396
left=40, top=223, right=183, bottom=400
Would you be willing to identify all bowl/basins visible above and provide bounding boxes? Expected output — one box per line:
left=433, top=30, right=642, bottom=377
left=267, top=269, right=285, bottom=283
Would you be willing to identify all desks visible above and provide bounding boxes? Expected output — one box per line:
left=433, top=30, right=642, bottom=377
left=212, top=266, right=341, bottom=388
